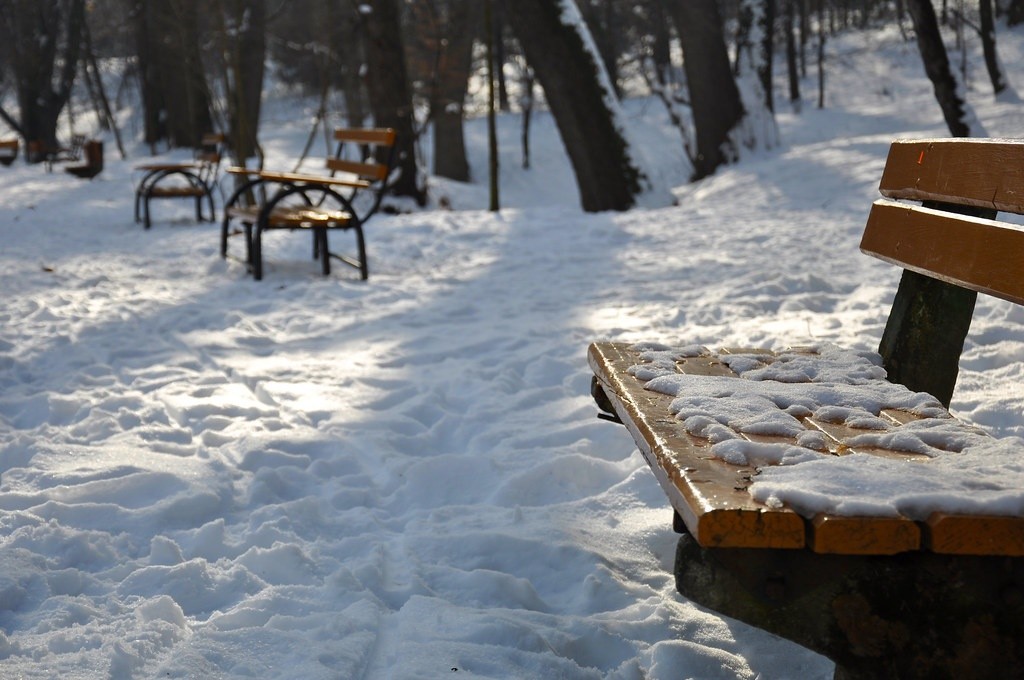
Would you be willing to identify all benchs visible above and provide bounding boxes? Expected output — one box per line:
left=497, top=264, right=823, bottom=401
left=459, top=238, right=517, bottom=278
left=0, top=139, right=19, bottom=168
left=133, top=132, right=227, bottom=230
left=45, top=132, right=85, bottom=171
left=222, top=127, right=397, bottom=281
left=586, top=138, right=1024, bottom=680
